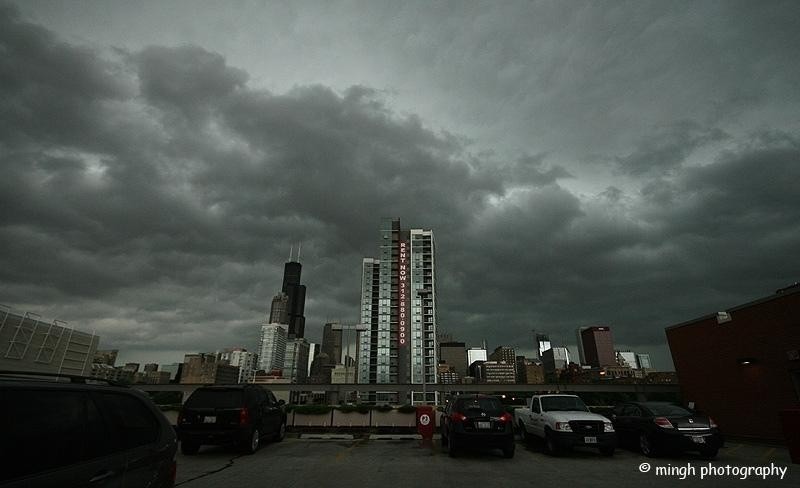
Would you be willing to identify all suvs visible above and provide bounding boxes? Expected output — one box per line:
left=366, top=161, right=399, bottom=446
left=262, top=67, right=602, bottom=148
left=1, top=369, right=176, bottom=487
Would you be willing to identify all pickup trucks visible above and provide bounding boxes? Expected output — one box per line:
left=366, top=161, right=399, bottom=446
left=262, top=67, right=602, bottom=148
left=515, top=394, right=615, bottom=457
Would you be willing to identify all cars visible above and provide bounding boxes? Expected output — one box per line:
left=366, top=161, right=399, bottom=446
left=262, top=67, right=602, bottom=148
left=616, top=401, right=721, bottom=458
left=178, top=383, right=288, bottom=454
left=437, top=395, right=516, bottom=458
